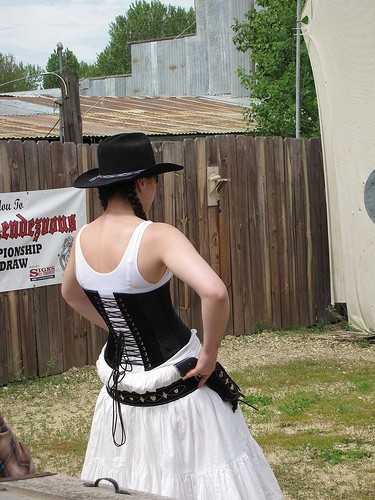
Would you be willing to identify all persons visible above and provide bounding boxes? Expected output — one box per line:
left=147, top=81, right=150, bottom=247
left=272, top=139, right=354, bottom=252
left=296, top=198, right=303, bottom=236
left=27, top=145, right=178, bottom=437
left=60, top=132, right=283, bottom=500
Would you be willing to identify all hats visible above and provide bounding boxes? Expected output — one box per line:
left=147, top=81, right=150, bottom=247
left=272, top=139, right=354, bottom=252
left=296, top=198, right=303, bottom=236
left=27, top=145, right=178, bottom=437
left=74, top=132, right=184, bottom=189
